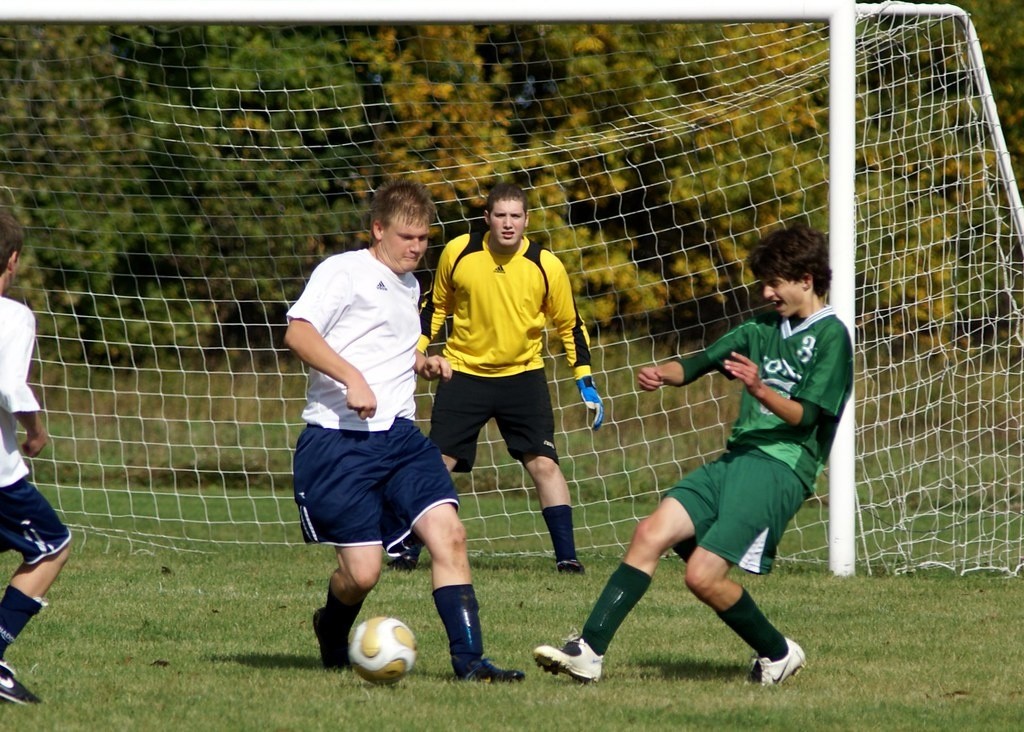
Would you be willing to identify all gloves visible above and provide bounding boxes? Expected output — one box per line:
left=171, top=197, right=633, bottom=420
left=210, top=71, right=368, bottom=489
left=576, top=375, right=604, bottom=431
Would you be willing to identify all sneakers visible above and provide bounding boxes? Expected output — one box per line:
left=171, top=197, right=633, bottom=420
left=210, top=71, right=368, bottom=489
left=313, top=606, right=352, bottom=674
left=746, top=637, right=806, bottom=689
left=457, top=656, right=525, bottom=682
left=0, top=660, right=41, bottom=705
left=534, top=635, right=603, bottom=684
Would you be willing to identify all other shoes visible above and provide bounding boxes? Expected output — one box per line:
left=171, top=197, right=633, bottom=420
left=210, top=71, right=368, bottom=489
left=557, top=558, right=585, bottom=575
left=387, top=547, right=419, bottom=572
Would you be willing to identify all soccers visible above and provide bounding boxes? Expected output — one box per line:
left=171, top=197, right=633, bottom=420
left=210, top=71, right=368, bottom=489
left=347, top=616, right=420, bottom=686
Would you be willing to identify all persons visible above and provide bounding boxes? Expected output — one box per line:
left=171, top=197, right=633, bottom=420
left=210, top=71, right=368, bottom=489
left=533, top=222, right=854, bottom=688
left=389, top=182, right=605, bottom=576
left=0, top=209, right=73, bottom=705
left=283, top=176, right=525, bottom=680
